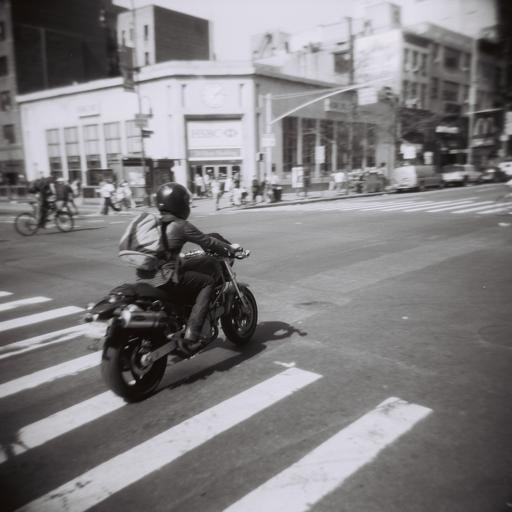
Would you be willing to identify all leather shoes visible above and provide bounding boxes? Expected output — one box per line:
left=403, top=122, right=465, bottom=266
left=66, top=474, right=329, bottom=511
left=183, top=328, right=212, bottom=344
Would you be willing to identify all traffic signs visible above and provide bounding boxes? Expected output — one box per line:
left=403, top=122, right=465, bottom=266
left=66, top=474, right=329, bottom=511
left=126, top=112, right=153, bottom=138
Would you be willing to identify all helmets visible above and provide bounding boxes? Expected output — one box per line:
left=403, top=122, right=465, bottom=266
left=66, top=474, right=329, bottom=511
left=154, top=182, right=192, bottom=220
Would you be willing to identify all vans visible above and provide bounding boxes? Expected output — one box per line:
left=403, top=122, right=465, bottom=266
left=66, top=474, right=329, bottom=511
left=441, top=163, right=481, bottom=187
left=390, top=165, right=440, bottom=192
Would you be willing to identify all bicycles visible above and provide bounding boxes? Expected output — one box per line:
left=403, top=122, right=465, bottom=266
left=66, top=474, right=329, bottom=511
left=44, top=192, right=79, bottom=221
left=14, top=196, right=74, bottom=237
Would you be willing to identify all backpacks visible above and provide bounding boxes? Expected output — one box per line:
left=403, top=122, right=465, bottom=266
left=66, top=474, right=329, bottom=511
left=117, top=211, right=185, bottom=270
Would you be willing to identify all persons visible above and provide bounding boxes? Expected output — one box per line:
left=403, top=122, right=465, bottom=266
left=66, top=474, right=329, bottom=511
left=194, top=173, right=268, bottom=210
left=297, top=164, right=311, bottom=197
left=28, top=173, right=79, bottom=228
left=135, top=183, right=243, bottom=343
left=328, top=166, right=379, bottom=196
left=98, top=177, right=132, bottom=215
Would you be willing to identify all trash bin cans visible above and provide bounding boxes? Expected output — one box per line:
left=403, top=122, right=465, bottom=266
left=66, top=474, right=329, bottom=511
left=272, top=187, right=283, bottom=201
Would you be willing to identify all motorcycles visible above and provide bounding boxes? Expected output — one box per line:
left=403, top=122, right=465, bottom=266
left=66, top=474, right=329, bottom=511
left=83, top=233, right=258, bottom=403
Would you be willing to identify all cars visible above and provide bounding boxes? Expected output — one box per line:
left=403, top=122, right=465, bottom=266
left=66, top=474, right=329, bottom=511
left=480, top=166, right=507, bottom=183
